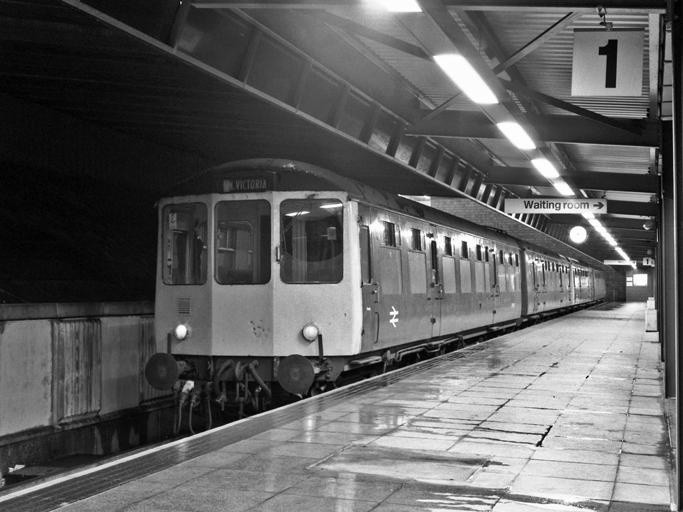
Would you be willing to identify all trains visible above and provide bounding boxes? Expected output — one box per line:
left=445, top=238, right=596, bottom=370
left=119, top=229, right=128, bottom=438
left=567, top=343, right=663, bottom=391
left=145, top=158, right=609, bottom=433
